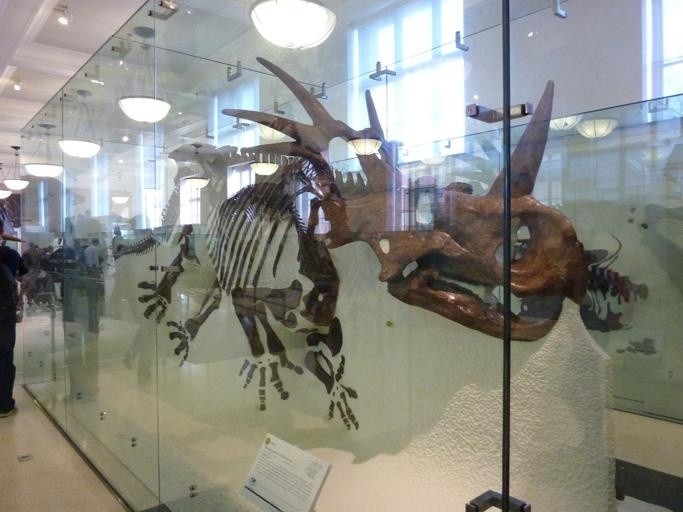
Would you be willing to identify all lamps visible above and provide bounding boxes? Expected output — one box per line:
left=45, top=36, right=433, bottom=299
left=117, top=26, right=172, bottom=123
left=109, top=172, right=130, bottom=205
left=183, top=144, right=209, bottom=189
left=248, top=134, right=279, bottom=176
left=246, top=0, right=338, bottom=51
left=0, top=90, right=101, bottom=200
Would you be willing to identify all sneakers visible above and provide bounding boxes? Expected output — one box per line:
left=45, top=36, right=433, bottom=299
left=2, top=407, right=17, bottom=418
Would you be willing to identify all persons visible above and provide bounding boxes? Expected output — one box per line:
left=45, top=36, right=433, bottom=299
left=0, top=218, right=29, bottom=420
left=30, top=214, right=156, bottom=404
